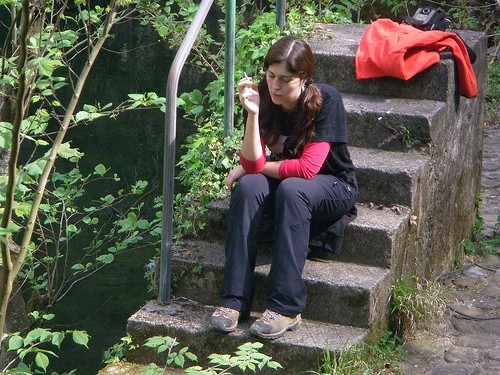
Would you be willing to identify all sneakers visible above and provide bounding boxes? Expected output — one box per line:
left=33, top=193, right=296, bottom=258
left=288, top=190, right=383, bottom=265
left=211, top=307, right=240, bottom=331
left=249, top=308, right=303, bottom=340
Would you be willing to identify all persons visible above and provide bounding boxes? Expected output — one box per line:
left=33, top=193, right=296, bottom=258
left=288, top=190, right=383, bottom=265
left=208, top=37, right=359, bottom=340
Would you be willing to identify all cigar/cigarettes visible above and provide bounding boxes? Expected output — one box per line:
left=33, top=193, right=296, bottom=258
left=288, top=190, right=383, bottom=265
left=243, top=71, right=249, bottom=81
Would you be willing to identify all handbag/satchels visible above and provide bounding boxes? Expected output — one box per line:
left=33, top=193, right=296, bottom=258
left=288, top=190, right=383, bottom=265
left=410, top=6, right=449, bottom=33
